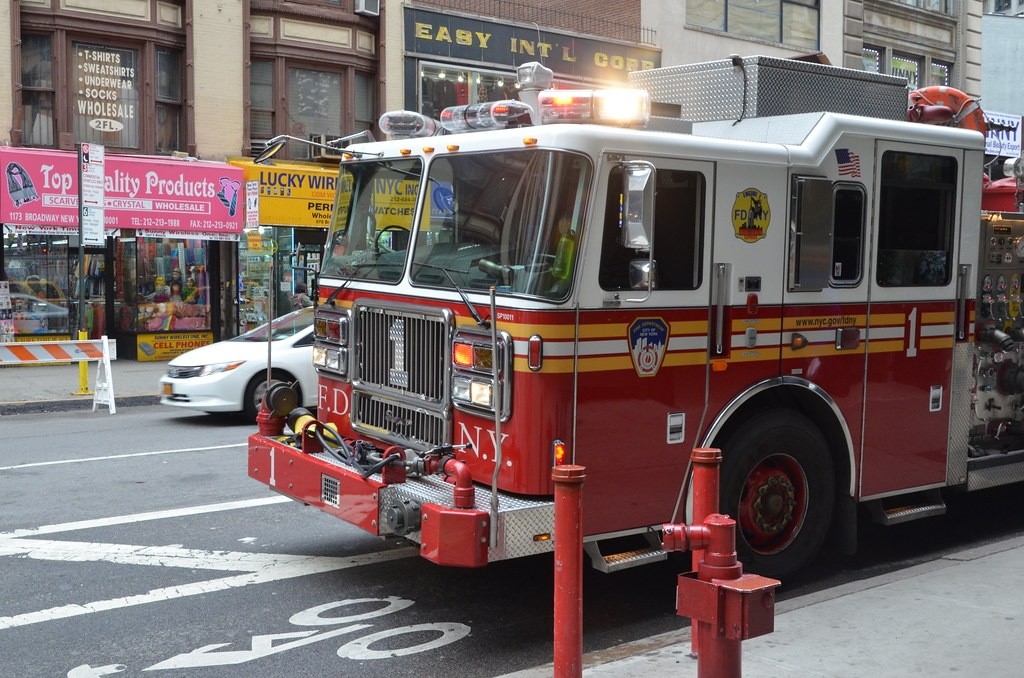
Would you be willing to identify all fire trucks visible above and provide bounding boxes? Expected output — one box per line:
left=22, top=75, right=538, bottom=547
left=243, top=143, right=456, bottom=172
left=245, top=52, right=1024, bottom=597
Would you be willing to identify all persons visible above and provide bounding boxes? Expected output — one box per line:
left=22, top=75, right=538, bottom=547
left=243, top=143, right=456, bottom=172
left=557, top=205, right=584, bottom=235
left=289, top=284, right=314, bottom=311
left=145, top=268, right=196, bottom=304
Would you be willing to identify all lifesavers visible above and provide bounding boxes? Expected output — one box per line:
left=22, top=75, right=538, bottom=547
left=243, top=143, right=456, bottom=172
left=911, top=82, right=986, bottom=137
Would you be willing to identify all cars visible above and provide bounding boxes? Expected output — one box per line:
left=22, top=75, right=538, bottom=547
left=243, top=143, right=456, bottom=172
left=160, top=306, right=318, bottom=425
left=10, top=293, right=69, bottom=331
